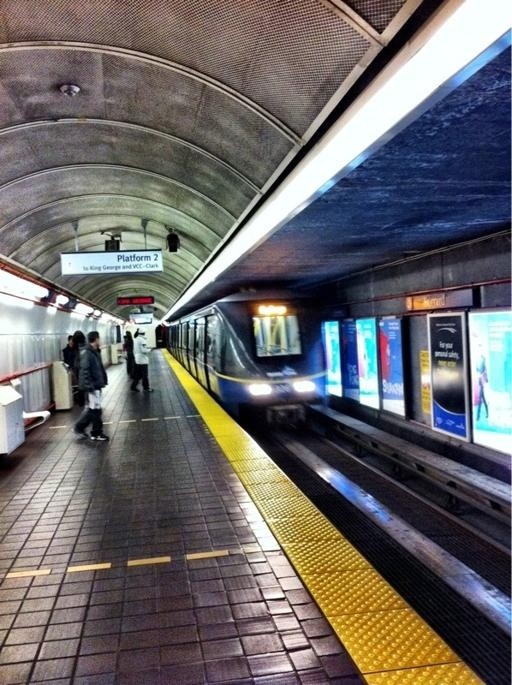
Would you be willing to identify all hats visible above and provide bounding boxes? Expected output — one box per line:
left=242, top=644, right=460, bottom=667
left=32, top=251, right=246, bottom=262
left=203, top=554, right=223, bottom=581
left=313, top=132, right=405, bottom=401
left=136, top=327, right=146, bottom=334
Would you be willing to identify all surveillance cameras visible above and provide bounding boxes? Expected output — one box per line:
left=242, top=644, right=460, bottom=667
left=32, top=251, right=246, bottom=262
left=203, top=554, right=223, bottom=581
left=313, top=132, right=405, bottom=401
left=60, top=85, right=80, bottom=100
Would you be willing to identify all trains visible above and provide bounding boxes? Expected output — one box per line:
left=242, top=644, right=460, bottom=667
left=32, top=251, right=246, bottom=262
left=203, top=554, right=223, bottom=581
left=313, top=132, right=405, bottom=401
left=158, top=289, right=326, bottom=428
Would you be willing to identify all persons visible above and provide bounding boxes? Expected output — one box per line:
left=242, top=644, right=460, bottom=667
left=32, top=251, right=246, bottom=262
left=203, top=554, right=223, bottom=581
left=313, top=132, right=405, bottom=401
left=70, top=331, right=87, bottom=415
left=474, top=352, right=493, bottom=421
left=72, top=331, right=109, bottom=441
left=63, top=335, right=76, bottom=363
left=122, top=329, right=154, bottom=392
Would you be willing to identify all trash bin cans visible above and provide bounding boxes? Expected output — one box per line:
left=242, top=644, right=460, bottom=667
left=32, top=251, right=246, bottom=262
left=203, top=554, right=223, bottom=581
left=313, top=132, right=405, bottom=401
left=110, top=344, right=119, bottom=365
left=53, top=360, right=74, bottom=410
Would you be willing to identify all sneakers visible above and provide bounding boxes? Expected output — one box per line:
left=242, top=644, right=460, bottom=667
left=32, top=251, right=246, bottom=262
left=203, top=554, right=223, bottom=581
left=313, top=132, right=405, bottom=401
left=131, top=384, right=154, bottom=392
left=71, top=426, right=109, bottom=440
